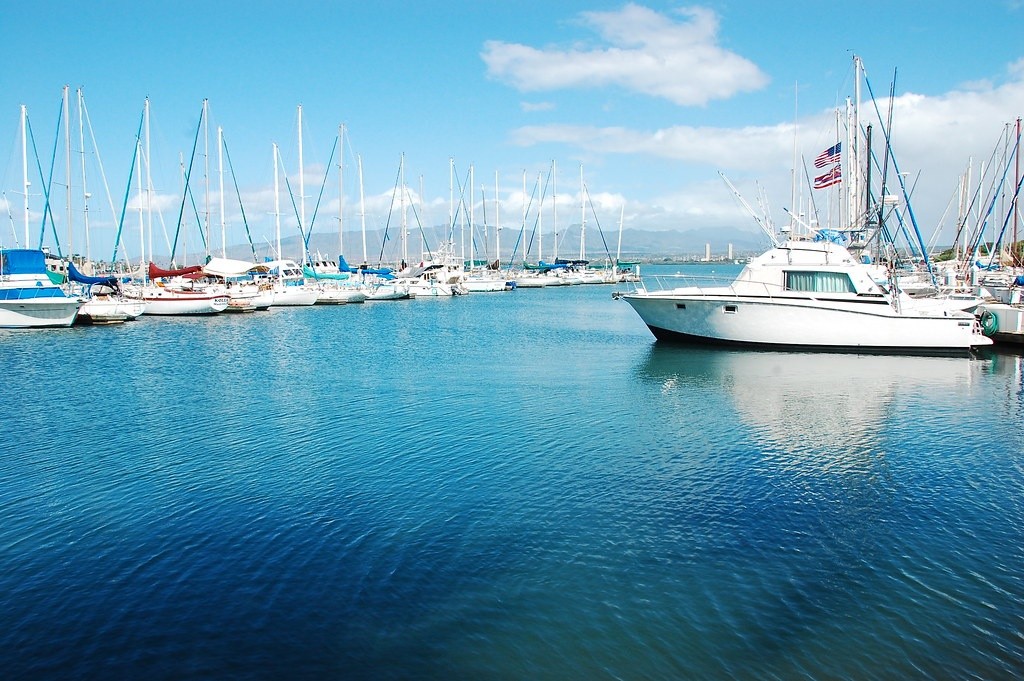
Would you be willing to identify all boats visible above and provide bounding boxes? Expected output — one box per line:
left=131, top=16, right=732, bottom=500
left=612, top=80, right=993, bottom=354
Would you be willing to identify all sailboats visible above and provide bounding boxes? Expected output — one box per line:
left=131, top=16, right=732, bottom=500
left=717, top=48, right=1024, bottom=345
left=0, top=85, right=638, bottom=331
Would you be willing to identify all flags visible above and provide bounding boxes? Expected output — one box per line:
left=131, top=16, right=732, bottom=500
left=814, top=142, right=841, bottom=168
left=813, top=163, right=842, bottom=190
